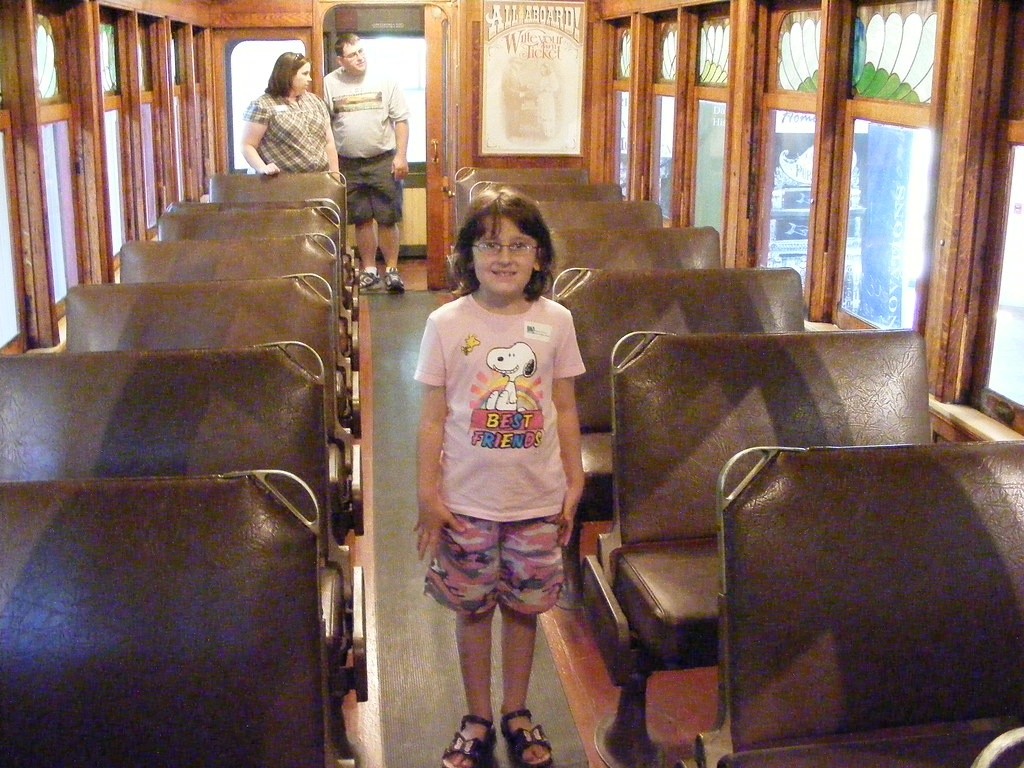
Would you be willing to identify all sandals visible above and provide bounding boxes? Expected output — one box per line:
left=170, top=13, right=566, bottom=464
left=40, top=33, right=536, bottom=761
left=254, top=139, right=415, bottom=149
left=500, top=710, right=553, bottom=768
left=442, top=714, right=497, bottom=768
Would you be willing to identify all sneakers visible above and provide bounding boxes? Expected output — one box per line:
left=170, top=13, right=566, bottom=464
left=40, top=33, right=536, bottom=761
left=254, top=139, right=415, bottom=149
left=358, top=268, right=382, bottom=295
left=382, top=267, right=405, bottom=293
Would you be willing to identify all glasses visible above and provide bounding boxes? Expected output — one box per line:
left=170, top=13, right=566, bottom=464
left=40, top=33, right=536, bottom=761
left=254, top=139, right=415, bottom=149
left=340, top=48, right=365, bottom=58
left=472, top=241, right=539, bottom=255
left=292, top=53, right=304, bottom=65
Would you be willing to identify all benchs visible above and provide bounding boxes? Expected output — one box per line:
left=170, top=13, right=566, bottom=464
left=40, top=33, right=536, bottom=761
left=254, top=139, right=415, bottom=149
left=0, top=171, right=369, bottom=768
left=447, top=166, right=1024, bottom=768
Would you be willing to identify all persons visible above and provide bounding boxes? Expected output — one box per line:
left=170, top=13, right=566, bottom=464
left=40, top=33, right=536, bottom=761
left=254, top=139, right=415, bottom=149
left=322, top=32, right=410, bottom=295
left=413, top=186, right=588, bottom=768
left=241, top=52, right=341, bottom=183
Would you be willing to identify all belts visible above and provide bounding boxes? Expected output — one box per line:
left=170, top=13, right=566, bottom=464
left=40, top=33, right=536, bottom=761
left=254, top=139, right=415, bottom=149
left=338, top=150, right=393, bottom=165
